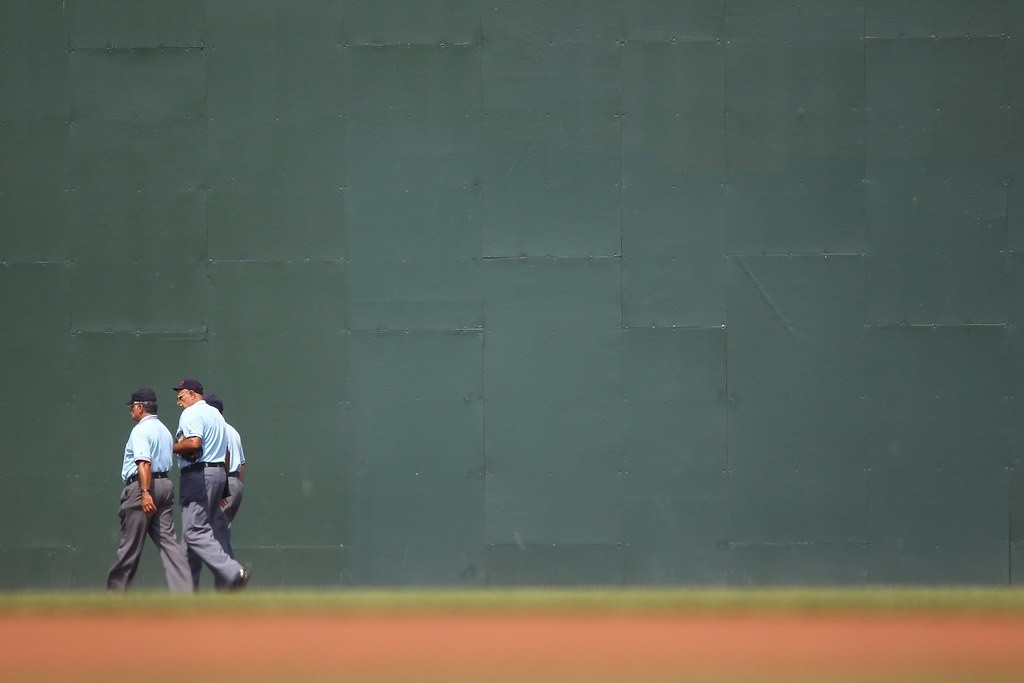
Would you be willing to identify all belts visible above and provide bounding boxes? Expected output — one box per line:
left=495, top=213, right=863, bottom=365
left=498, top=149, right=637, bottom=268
left=180, top=461, right=225, bottom=475
left=229, top=470, right=241, bottom=477
left=124, top=471, right=168, bottom=483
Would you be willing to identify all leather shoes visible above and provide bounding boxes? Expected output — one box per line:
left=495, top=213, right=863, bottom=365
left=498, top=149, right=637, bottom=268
left=228, top=567, right=252, bottom=590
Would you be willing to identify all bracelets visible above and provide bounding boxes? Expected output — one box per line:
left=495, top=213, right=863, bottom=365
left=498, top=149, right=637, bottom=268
left=141, top=489, right=150, bottom=492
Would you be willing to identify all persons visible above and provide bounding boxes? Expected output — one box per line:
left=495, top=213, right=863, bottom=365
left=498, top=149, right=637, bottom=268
left=107, top=379, right=251, bottom=593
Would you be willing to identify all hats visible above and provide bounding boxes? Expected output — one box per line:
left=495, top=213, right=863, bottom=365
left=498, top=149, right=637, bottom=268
left=171, top=379, right=204, bottom=397
left=204, top=393, right=224, bottom=414
left=125, top=388, right=157, bottom=407
